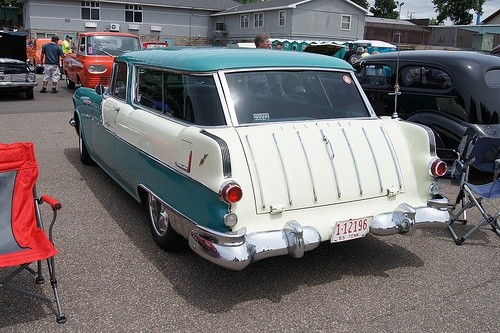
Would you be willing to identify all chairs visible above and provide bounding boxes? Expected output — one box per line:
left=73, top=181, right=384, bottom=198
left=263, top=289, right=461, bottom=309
left=447, top=136, right=500, bottom=246
left=185, top=93, right=218, bottom=123
left=0, top=140, right=67, bottom=324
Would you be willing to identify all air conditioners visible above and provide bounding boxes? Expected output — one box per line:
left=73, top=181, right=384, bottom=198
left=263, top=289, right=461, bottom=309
left=110, top=23, right=119, bottom=31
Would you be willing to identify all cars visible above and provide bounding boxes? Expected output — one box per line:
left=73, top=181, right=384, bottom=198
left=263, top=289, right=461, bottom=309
left=0, top=31, right=38, bottom=100
left=69, top=45, right=452, bottom=271
left=350, top=50, right=500, bottom=174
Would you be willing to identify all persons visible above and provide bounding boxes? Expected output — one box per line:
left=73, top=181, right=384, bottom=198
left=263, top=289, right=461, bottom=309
left=343, top=44, right=383, bottom=75
left=255, top=33, right=272, bottom=49
left=276, top=42, right=283, bottom=50
left=293, top=46, right=297, bottom=51
left=62, top=35, right=72, bottom=55
left=40, top=35, right=65, bottom=93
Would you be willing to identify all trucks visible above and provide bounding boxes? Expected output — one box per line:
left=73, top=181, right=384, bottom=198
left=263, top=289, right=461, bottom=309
left=63, top=32, right=140, bottom=89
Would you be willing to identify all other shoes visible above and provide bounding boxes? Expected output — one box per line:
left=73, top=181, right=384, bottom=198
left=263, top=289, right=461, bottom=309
left=40, top=88, right=46, bottom=92
left=52, top=88, right=57, bottom=92
